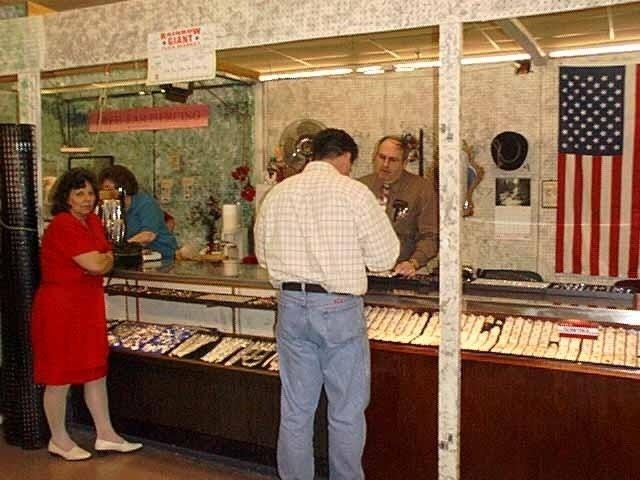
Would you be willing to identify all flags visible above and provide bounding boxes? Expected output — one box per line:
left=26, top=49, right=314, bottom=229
left=554, top=59, right=640, bottom=280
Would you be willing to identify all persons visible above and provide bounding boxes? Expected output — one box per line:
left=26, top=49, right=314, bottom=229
left=96, top=163, right=179, bottom=266
left=26, top=166, right=145, bottom=462
left=352, top=133, right=442, bottom=285
left=250, top=126, right=407, bottom=480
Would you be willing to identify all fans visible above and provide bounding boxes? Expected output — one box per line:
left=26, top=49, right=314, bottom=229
left=279, top=119, right=327, bottom=169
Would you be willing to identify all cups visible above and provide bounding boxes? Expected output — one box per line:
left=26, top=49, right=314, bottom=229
left=223, top=260, right=238, bottom=277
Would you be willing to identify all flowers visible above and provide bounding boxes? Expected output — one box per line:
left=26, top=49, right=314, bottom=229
left=233, top=165, right=255, bottom=207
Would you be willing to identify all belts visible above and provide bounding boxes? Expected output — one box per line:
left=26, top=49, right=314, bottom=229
left=282, top=281, right=352, bottom=296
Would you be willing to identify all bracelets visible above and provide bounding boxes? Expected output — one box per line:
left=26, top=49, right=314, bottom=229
left=408, top=260, right=418, bottom=271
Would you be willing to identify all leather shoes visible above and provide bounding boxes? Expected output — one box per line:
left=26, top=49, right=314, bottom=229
left=94, top=438, right=144, bottom=457
left=47, top=438, right=92, bottom=460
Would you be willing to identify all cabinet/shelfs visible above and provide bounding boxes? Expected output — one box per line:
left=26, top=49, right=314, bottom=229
left=101, top=257, right=324, bottom=476
left=328, top=268, right=640, bottom=480
